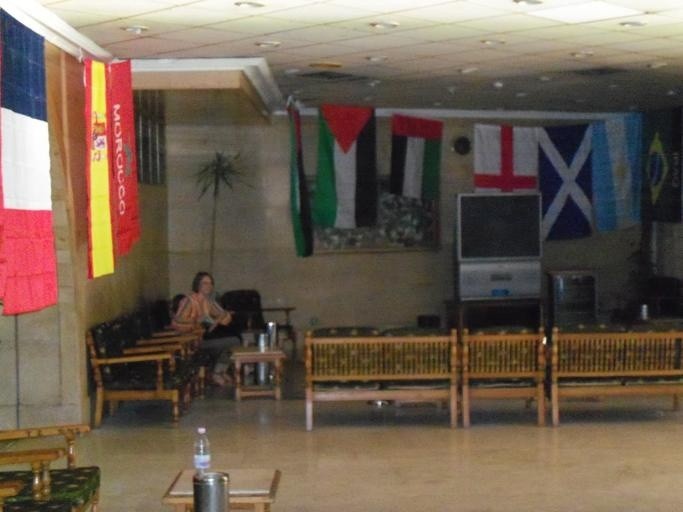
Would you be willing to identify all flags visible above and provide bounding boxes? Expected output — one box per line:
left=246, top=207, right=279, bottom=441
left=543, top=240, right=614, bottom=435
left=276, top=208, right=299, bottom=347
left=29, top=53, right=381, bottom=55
left=82, top=58, right=117, bottom=280
left=541, top=119, right=594, bottom=240
left=1, top=8, right=59, bottom=315
left=641, top=104, right=683, bottom=223
left=108, top=57, right=141, bottom=258
left=595, top=113, right=642, bottom=233
left=473, top=123, right=540, bottom=193
left=389, top=112, right=444, bottom=201
left=286, top=101, right=313, bottom=256
left=312, top=103, right=378, bottom=228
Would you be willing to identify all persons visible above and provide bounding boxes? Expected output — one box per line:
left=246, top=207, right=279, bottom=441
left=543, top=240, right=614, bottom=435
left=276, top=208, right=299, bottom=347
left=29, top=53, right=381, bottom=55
left=172, top=271, right=241, bottom=386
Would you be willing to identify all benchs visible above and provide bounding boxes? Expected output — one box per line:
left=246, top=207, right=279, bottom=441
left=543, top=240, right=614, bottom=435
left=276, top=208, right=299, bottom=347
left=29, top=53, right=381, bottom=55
left=551, top=325, right=681, bottom=428
left=307, top=323, right=459, bottom=428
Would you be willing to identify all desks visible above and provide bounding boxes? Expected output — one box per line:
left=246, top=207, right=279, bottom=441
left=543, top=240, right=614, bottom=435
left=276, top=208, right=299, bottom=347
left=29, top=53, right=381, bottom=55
left=444, top=298, right=546, bottom=330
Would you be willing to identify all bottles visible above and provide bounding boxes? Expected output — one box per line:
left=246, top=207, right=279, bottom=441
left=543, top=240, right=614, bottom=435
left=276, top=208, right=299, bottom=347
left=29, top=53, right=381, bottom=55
left=191, top=472, right=229, bottom=512
left=192, top=428, right=212, bottom=477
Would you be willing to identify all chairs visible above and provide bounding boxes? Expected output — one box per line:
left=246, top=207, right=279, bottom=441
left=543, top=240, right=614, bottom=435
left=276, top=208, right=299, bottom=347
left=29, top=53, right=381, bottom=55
left=2, top=426, right=103, bottom=510
left=88, top=288, right=299, bottom=428
left=462, top=327, right=548, bottom=425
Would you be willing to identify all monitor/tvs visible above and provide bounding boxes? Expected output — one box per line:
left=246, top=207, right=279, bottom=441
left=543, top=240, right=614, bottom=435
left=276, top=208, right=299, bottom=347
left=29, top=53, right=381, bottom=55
left=456, top=189, right=544, bottom=262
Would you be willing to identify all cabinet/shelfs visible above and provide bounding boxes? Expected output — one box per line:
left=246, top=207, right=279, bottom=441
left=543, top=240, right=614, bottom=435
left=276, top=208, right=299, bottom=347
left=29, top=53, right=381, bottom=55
left=547, top=267, right=604, bottom=323
